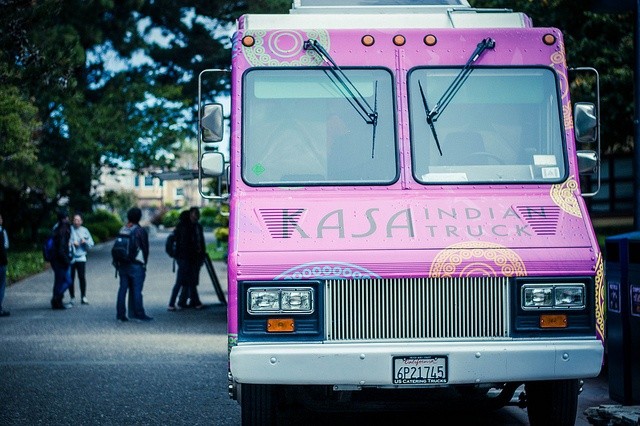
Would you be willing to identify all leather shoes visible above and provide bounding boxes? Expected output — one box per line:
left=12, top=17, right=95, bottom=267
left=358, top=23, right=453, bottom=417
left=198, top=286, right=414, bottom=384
left=53, top=302, right=72, bottom=309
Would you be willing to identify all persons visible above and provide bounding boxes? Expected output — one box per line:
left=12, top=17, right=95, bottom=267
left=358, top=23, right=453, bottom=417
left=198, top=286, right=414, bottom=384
left=114, top=207, right=153, bottom=321
left=167, top=211, right=203, bottom=312
left=178, top=208, right=202, bottom=309
left=70, top=214, right=94, bottom=304
left=49, top=209, right=74, bottom=309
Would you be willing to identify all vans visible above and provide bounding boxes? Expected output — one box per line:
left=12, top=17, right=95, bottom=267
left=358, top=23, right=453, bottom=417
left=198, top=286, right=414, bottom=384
left=195, top=0, right=600, bottom=425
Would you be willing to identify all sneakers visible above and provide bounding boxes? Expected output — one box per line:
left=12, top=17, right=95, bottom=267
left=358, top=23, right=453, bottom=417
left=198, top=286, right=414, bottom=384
left=71, top=297, right=76, bottom=305
left=129, top=315, right=154, bottom=323
left=116, top=314, right=129, bottom=322
left=0, top=310, right=11, bottom=317
left=81, top=296, right=90, bottom=305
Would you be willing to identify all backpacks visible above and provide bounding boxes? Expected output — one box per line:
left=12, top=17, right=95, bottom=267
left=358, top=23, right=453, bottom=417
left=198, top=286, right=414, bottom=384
left=111, top=225, right=140, bottom=278
left=43, top=222, right=68, bottom=262
left=165, top=223, right=183, bottom=273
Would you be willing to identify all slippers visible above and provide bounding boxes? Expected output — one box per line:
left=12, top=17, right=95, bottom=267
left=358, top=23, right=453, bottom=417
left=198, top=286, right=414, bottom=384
left=167, top=305, right=182, bottom=311
left=197, top=304, right=209, bottom=310
